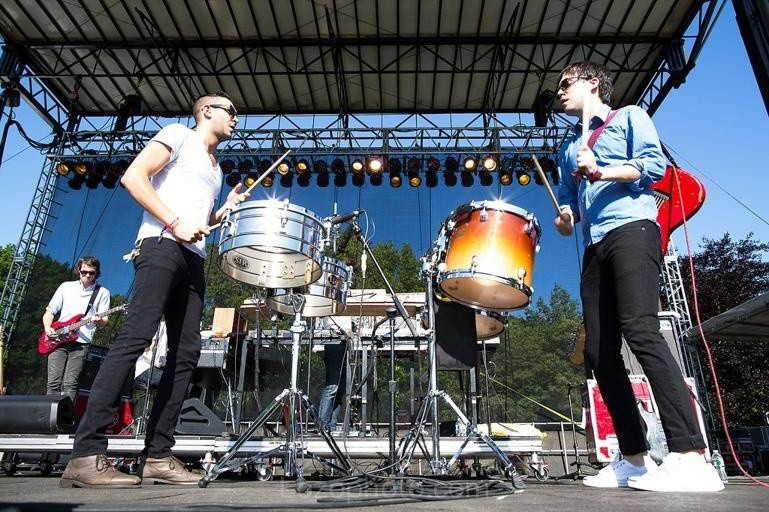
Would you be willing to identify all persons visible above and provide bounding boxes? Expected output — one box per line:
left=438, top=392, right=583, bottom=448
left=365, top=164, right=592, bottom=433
left=42, top=256, right=111, bottom=410
left=58, top=91, right=252, bottom=489
left=318, top=258, right=359, bottom=437
left=552, top=61, right=725, bottom=494
left=134, top=318, right=193, bottom=433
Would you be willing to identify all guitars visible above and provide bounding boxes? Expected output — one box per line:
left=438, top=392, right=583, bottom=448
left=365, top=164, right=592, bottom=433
left=37, top=303, right=129, bottom=355
left=569, top=166, right=708, bottom=366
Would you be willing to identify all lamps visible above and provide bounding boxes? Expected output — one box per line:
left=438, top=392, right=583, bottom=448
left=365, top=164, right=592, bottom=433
left=56, top=156, right=559, bottom=190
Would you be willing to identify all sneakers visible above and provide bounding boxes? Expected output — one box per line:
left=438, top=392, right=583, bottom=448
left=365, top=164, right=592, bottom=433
left=627, top=452, right=725, bottom=492
left=583, top=460, right=659, bottom=488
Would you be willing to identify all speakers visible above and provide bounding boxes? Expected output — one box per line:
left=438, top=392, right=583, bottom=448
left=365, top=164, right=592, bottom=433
left=0, top=394, right=81, bottom=435
left=432, top=297, right=478, bottom=371
left=619, top=316, right=688, bottom=377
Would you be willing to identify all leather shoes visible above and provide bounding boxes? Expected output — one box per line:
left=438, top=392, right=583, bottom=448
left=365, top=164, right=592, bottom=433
left=137, top=456, right=205, bottom=485
left=58, top=454, right=142, bottom=488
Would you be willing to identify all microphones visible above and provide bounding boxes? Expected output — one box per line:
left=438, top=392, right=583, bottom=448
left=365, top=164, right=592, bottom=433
left=337, top=222, right=354, bottom=253
left=332, top=209, right=363, bottom=223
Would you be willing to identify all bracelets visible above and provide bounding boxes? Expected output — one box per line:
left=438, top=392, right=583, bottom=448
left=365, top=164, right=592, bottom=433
left=159, top=216, right=181, bottom=242
left=586, top=165, right=603, bottom=183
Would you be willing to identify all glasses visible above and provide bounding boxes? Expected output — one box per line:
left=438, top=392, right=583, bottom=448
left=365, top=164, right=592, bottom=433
left=202, top=105, right=235, bottom=117
left=80, top=271, right=95, bottom=275
left=555, top=77, right=591, bottom=101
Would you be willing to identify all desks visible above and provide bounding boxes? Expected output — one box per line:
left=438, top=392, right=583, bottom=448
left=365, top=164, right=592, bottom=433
left=240, top=291, right=500, bottom=436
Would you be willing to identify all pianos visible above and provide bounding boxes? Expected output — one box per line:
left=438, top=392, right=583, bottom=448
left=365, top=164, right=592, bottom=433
left=159, top=339, right=293, bottom=374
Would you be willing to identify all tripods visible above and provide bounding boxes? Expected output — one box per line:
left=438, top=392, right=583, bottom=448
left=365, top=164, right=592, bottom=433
left=554, top=382, right=601, bottom=481
left=399, top=274, right=522, bottom=489
left=200, top=296, right=352, bottom=492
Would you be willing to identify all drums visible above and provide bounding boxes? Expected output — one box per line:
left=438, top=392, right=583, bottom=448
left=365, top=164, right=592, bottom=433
left=266, top=256, right=353, bottom=319
left=217, top=200, right=326, bottom=288
left=436, top=200, right=541, bottom=312
left=421, top=312, right=509, bottom=342
